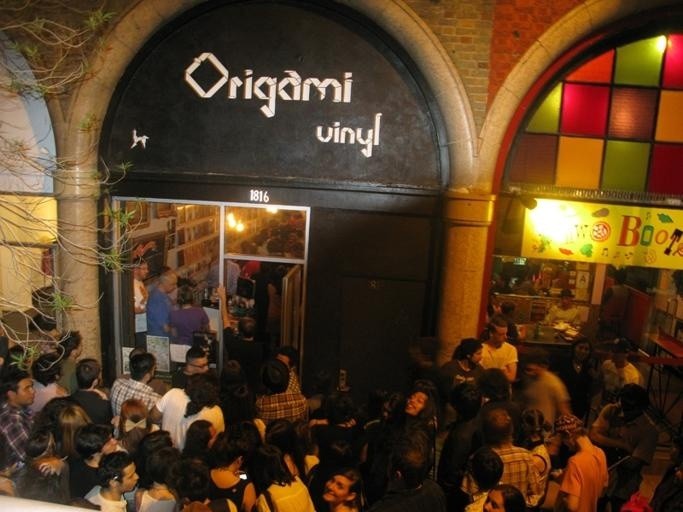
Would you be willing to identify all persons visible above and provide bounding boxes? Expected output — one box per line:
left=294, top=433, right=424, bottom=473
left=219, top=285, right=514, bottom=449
left=1, top=209, right=683, bottom=512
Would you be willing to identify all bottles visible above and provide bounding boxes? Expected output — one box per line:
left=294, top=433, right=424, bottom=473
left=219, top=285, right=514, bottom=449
left=201, top=288, right=210, bottom=307
left=535, top=322, right=541, bottom=339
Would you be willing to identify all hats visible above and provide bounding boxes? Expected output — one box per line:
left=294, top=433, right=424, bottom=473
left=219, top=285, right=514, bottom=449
left=554, top=413, right=581, bottom=432
left=558, top=290, right=574, bottom=297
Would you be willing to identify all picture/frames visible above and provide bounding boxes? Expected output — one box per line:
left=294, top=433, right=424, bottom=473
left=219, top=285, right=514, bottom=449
left=155, top=203, right=178, bottom=219
left=125, top=202, right=151, bottom=232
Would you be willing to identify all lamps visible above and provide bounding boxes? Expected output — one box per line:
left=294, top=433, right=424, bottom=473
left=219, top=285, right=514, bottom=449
left=518, top=194, right=537, bottom=209
